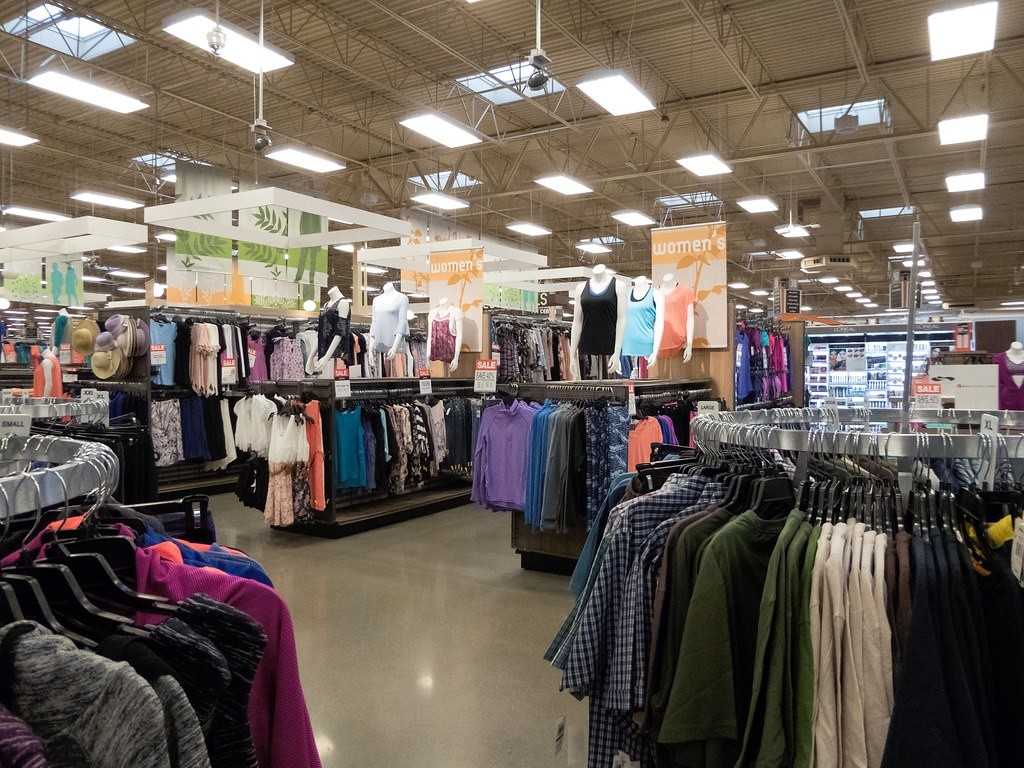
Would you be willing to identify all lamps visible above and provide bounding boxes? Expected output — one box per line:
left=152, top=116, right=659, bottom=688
left=2, top=1, right=1001, bottom=304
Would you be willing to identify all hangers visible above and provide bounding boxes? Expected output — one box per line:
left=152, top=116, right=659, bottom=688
left=0, top=314, right=1024, bottom=768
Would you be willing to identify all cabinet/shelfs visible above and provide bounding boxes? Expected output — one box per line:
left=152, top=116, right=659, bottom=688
left=1, top=396, right=108, bottom=417
left=62, top=300, right=430, bottom=524
left=1, top=336, right=52, bottom=390
left=805, top=321, right=975, bottom=434
left=889, top=352, right=998, bottom=428
left=244, top=305, right=649, bottom=538
left=494, top=299, right=806, bottom=576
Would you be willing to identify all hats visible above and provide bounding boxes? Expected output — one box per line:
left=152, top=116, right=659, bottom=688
left=114, top=318, right=150, bottom=357
left=94, top=331, right=114, bottom=351
left=91, top=347, right=133, bottom=380
left=71, top=319, right=101, bottom=357
left=104, top=314, right=129, bottom=339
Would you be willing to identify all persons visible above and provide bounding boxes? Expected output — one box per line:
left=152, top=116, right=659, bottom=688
left=622, top=263, right=696, bottom=377
left=33, top=348, right=63, bottom=397
left=931, top=348, right=942, bottom=365
left=52, top=308, right=73, bottom=356
left=368, top=283, right=409, bottom=366
left=830, top=349, right=846, bottom=371
left=427, top=297, right=464, bottom=373
left=305, top=286, right=351, bottom=375
left=569, top=264, right=628, bottom=377
left=991, top=341, right=1024, bottom=411
